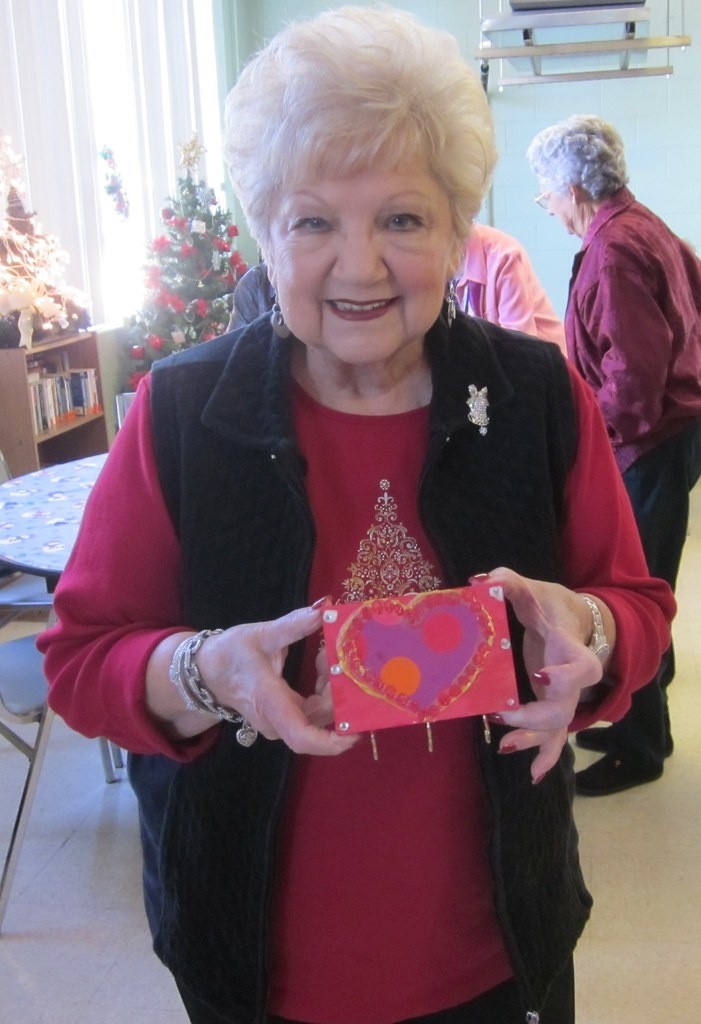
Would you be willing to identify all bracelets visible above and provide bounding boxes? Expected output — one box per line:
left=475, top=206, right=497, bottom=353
left=170, top=628, right=259, bottom=746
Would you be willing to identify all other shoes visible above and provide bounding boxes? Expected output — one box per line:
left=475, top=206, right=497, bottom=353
left=574, top=753, right=663, bottom=795
left=576, top=727, right=616, bottom=753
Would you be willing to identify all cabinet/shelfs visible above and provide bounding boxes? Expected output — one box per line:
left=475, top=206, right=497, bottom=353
left=0, top=330, right=111, bottom=480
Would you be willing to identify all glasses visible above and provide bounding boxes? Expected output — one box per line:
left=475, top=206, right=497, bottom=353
left=533, top=190, right=552, bottom=210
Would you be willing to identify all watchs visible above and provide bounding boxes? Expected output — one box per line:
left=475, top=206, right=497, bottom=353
left=584, top=598, right=610, bottom=670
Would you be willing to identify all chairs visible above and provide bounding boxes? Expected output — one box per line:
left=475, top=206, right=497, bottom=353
left=0, top=632, right=119, bottom=935
left=0, top=572, right=60, bottom=631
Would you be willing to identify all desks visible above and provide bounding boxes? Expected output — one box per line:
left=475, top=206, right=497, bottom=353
left=0, top=451, right=110, bottom=573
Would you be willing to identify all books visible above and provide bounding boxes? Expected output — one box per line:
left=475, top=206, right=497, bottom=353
left=27, top=350, right=99, bottom=435
left=114, top=392, right=136, bottom=433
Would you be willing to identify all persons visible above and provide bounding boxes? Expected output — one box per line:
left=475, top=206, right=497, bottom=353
left=36, top=6, right=677, bottom=1024
left=222, top=259, right=280, bottom=334
left=527, top=111, right=701, bottom=797
left=446, top=223, right=567, bottom=357
